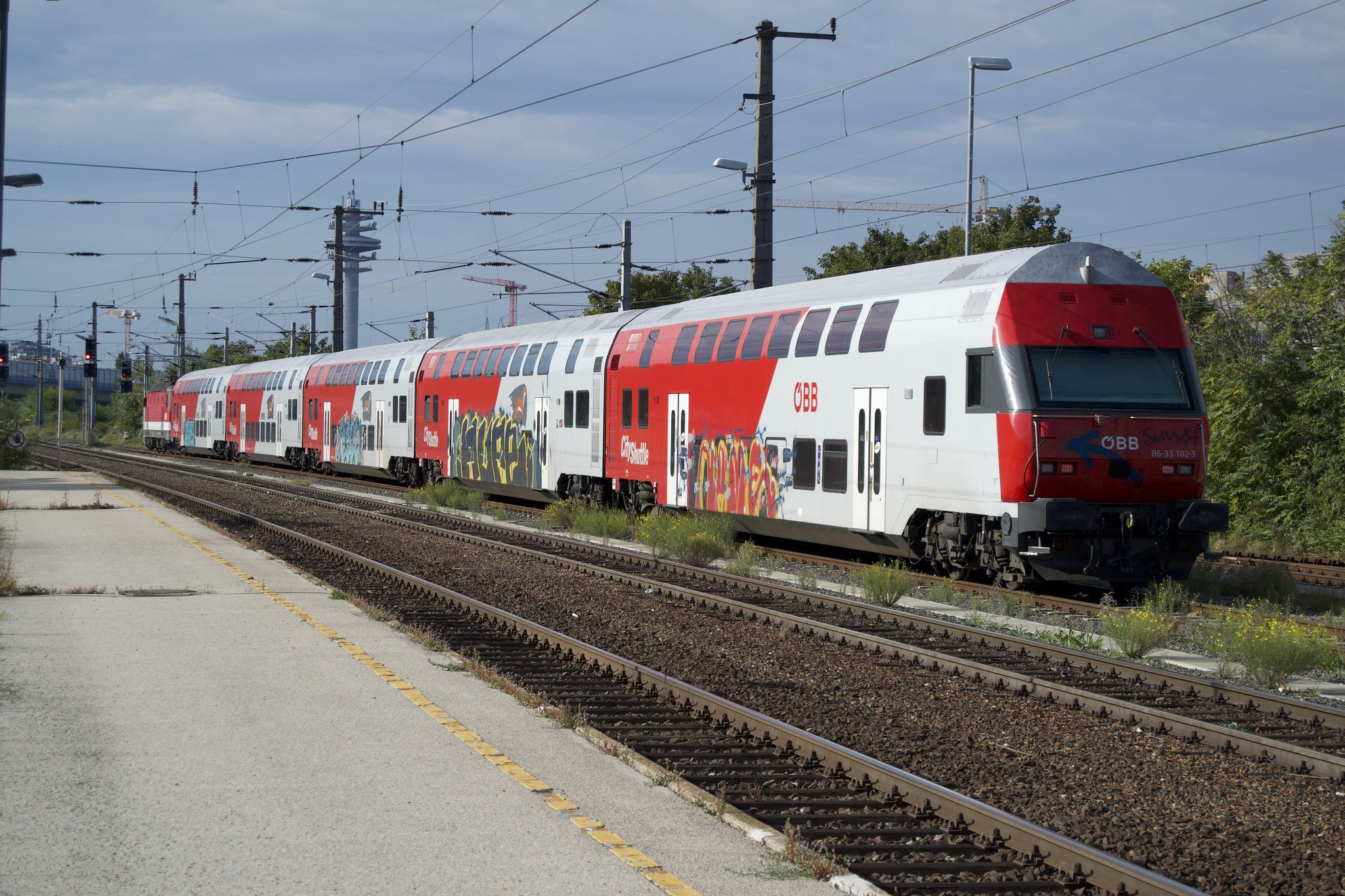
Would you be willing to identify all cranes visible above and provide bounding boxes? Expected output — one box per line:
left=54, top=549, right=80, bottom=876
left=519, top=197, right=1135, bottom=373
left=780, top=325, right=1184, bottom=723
left=462, top=275, right=527, bottom=327
left=104, top=309, right=142, bottom=359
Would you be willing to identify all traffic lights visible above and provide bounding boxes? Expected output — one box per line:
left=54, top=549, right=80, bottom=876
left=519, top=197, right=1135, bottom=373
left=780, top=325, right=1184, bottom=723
left=84, top=363, right=98, bottom=378
left=85, top=339, right=94, bottom=360
left=121, top=380, right=133, bottom=394
left=0, top=344, right=9, bottom=363
left=121, top=359, right=132, bottom=377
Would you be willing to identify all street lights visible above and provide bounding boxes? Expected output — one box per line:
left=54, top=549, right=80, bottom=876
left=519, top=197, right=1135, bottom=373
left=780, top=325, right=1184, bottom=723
left=311, top=272, right=343, bottom=354
left=712, top=158, right=772, bottom=290
left=965, top=58, right=1013, bottom=255
left=156, top=315, right=186, bottom=378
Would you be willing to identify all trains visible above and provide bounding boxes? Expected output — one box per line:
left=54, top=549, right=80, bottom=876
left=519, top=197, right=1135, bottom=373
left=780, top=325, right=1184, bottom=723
left=146, top=241, right=1230, bottom=598
